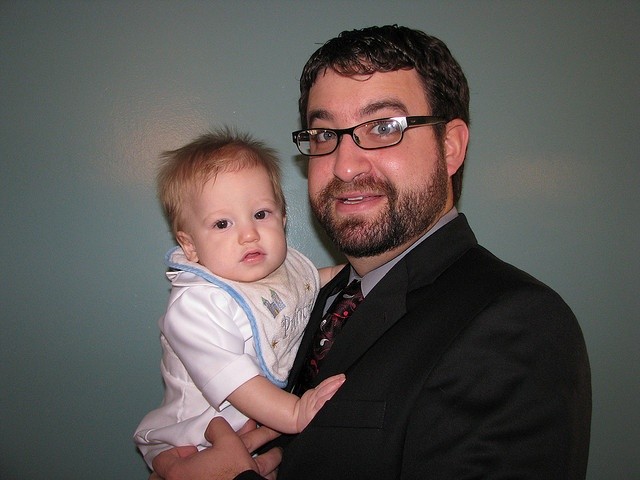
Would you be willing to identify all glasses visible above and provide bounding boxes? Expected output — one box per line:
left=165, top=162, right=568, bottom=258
left=292, top=116, right=450, bottom=157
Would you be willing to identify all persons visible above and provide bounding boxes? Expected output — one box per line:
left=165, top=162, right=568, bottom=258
left=148, top=24, right=593, bottom=480
left=132, top=122, right=346, bottom=471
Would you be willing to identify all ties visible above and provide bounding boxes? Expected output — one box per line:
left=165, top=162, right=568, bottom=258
left=296, top=279, right=364, bottom=383
